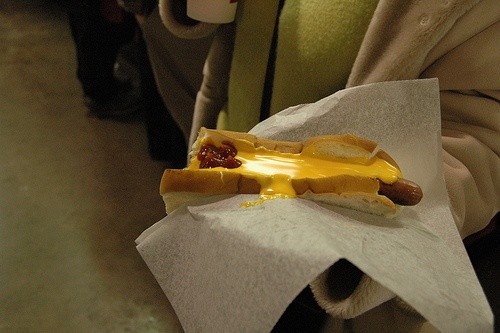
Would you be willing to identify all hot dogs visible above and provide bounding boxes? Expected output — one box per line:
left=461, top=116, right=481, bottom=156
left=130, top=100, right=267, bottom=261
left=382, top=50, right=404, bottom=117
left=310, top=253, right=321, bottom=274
left=160, top=127, right=425, bottom=218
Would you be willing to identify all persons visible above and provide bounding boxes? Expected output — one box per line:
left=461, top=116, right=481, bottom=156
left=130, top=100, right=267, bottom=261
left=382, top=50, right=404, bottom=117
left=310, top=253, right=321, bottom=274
left=64, top=0, right=214, bottom=171
left=159, top=0, right=499, bottom=333
